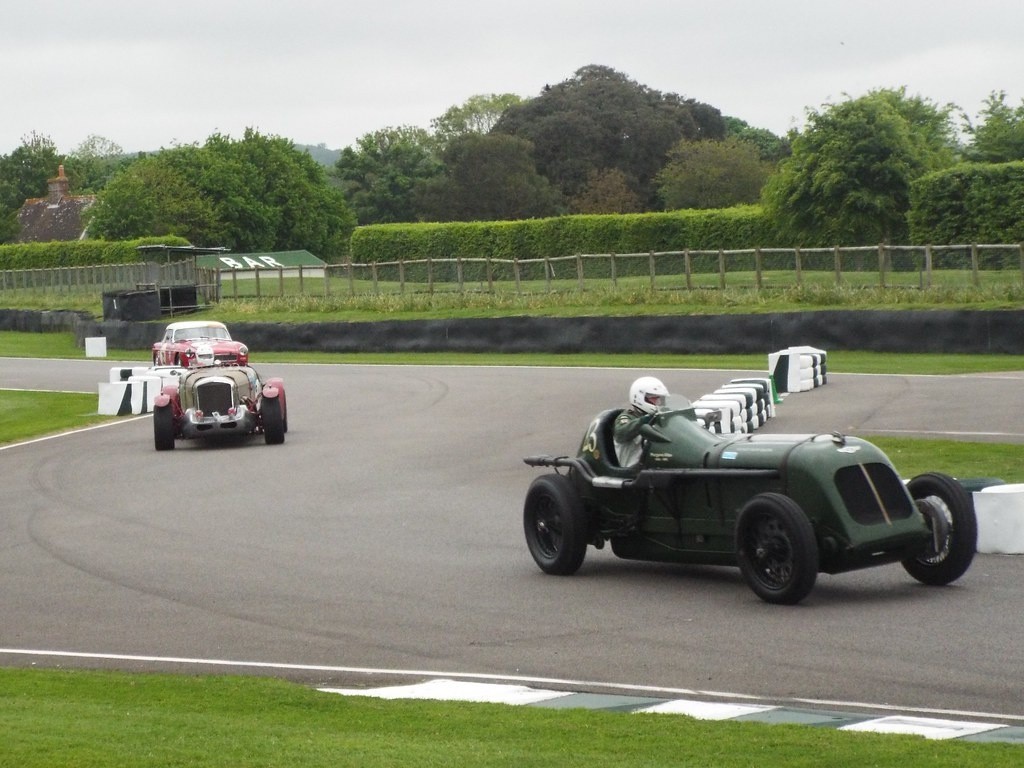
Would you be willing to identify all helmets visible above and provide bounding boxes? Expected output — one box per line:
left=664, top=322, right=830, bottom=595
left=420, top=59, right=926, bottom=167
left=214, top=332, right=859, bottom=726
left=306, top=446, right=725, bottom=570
left=196, top=344, right=214, bottom=367
left=629, top=376, right=670, bottom=415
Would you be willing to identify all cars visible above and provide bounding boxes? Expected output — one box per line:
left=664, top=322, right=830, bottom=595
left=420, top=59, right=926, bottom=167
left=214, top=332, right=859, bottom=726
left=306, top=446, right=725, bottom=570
left=523, top=393, right=978, bottom=605
left=153, top=360, right=288, bottom=450
left=153, top=321, right=249, bottom=368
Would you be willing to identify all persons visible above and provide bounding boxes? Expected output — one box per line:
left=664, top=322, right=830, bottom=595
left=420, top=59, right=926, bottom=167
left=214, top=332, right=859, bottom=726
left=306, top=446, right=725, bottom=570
left=195, top=344, right=221, bottom=366
left=612, top=376, right=671, bottom=468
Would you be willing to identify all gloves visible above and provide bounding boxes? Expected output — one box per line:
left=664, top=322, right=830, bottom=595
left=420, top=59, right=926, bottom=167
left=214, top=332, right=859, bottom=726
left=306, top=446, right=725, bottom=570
left=650, top=406, right=657, bottom=415
